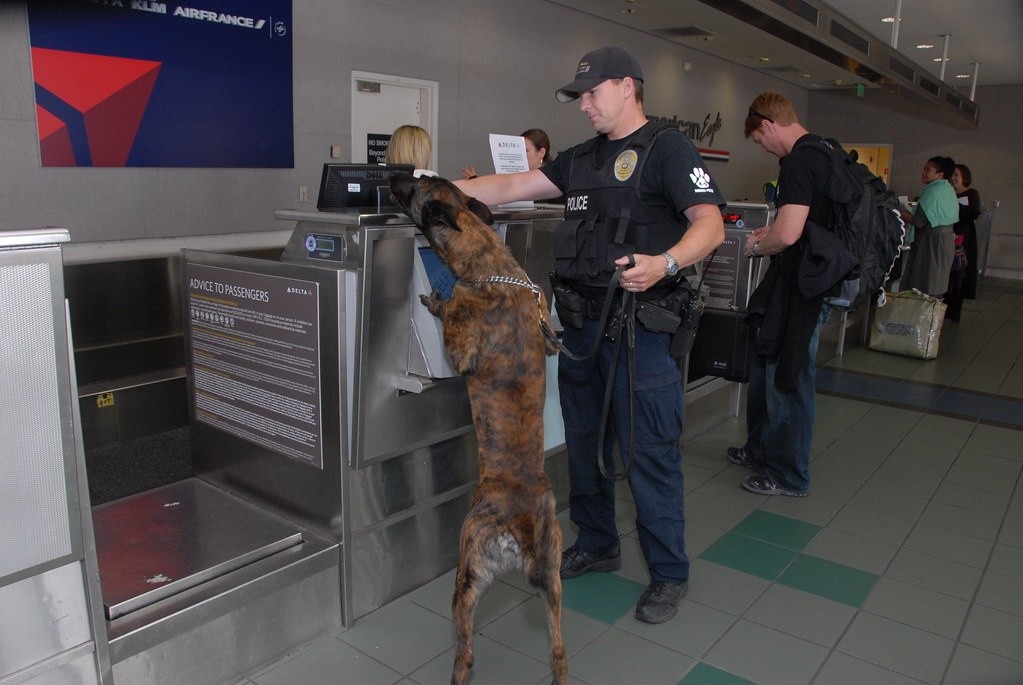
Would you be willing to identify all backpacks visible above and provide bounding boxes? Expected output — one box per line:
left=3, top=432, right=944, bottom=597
left=791, top=138, right=908, bottom=313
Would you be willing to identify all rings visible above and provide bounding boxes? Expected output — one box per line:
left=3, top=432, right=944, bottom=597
left=630, top=282, right=633, bottom=288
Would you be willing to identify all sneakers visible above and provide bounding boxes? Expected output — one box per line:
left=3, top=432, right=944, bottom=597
left=559, top=541, right=622, bottom=579
left=634, top=580, right=690, bottom=625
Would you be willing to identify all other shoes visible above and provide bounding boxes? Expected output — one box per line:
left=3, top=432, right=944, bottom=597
left=727, top=444, right=809, bottom=497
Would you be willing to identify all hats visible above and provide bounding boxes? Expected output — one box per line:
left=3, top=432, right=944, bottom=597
left=555, top=46, right=644, bottom=103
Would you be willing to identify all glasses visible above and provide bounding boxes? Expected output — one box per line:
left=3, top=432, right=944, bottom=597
left=747, top=106, right=774, bottom=123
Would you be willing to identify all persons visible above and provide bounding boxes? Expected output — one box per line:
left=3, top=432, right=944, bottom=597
left=942, top=164, right=983, bottom=324
left=385, top=125, right=436, bottom=173
left=461, top=128, right=566, bottom=206
left=897, top=155, right=961, bottom=311
left=448, top=46, right=730, bottom=625
left=725, top=91, right=863, bottom=498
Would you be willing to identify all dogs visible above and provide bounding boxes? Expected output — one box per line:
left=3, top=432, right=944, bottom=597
left=388, top=170, right=569, bottom=685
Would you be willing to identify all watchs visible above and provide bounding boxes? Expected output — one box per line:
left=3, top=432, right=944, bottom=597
left=751, top=240, right=760, bottom=256
left=661, top=252, right=679, bottom=276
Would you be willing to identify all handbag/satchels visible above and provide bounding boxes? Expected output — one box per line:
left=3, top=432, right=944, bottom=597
left=868, top=289, right=947, bottom=360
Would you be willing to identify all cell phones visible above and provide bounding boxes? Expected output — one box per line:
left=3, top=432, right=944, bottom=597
left=679, top=299, right=703, bottom=332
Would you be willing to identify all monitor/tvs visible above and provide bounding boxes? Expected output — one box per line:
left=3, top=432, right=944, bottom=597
left=316, top=163, right=416, bottom=211
